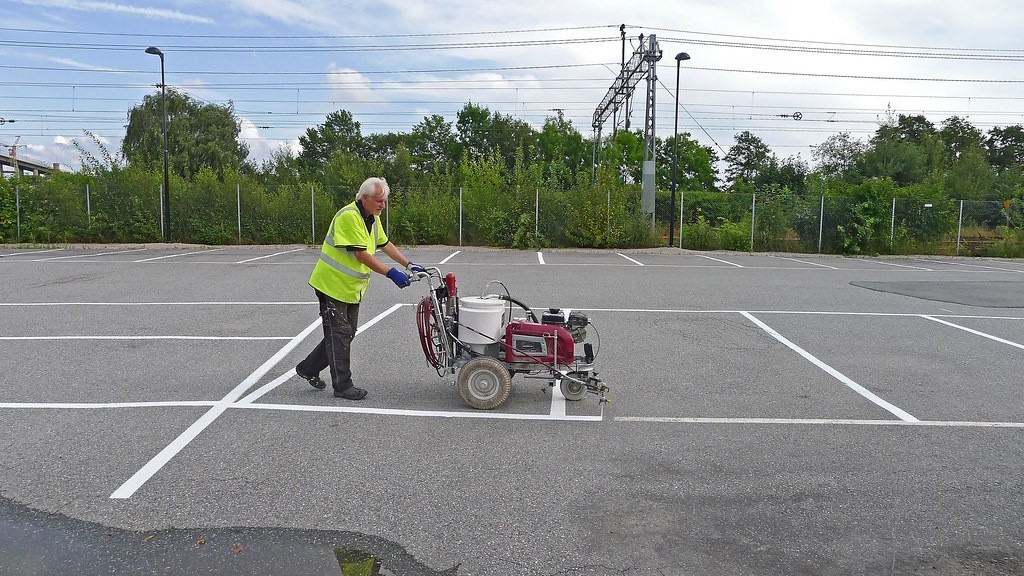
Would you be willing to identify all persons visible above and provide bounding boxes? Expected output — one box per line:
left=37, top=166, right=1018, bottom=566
left=296, top=177, right=427, bottom=401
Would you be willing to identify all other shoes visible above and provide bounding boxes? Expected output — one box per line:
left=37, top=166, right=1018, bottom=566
left=296, top=364, right=328, bottom=391
left=334, top=386, right=367, bottom=400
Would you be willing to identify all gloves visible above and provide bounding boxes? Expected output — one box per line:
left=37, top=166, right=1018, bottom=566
left=406, top=261, right=427, bottom=277
left=386, top=267, right=410, bottom=289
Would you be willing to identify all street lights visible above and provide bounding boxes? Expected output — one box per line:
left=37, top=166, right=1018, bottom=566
left=144, top=46, right=172, bottom=243
left=669, top=52, right=691, bottom=247
